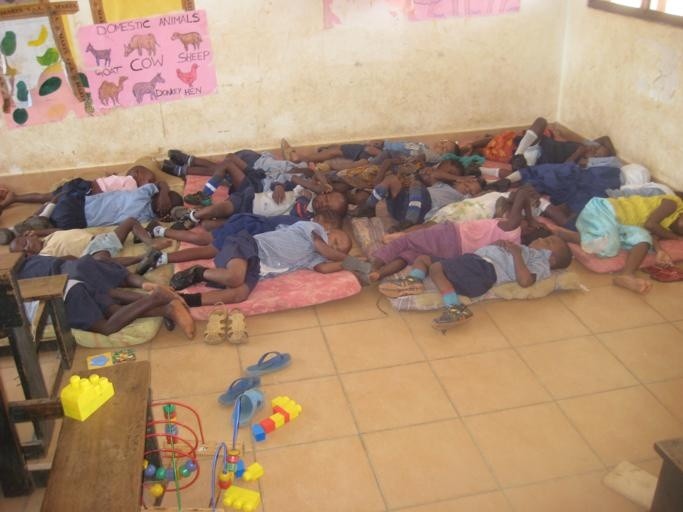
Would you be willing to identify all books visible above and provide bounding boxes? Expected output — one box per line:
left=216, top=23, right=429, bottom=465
left=111, top=347, right=135, bottom=362
left=86, top=352, right=112, bottom=369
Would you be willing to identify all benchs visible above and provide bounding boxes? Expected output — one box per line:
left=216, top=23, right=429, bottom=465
left=41, top=360, right=156, bottom=511
left=0, top=274, right=79, bottom=371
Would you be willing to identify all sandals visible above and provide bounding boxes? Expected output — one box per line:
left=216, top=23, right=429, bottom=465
left=228, top=306, right=248, bottom=346
left=203, top=301, right=227, bottom=344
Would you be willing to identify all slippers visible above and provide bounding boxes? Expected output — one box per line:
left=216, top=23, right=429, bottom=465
left=217, top=375, right=260, bottom=406
left=246, top=351, right=291, bottom=376
left=230, top=388, right=264, bottom=426
left=642, top=263, right=683, bottom=282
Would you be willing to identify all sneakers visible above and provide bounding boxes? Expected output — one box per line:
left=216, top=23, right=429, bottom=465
left=168, top=148, right=188, bottom=165
left=133, top=219, right=161, bottom=243
left=431, top=303, right=473, bottom=328
left=170, top=264, right=204, bottom=291
left=161, top=159, right=184, bottom=178
left=162, top=292, right=188, bottom=332
left=184, top=189, right=211, bottom=206
left=377, top=276, right=425, bottom=299
left=135, top=246, right=162, bottom=276
left=169, top=205, right=202, bottom=231
left=346, top=203, right=376, bottom=217
left=388, top=220, right=413, bottom=233
